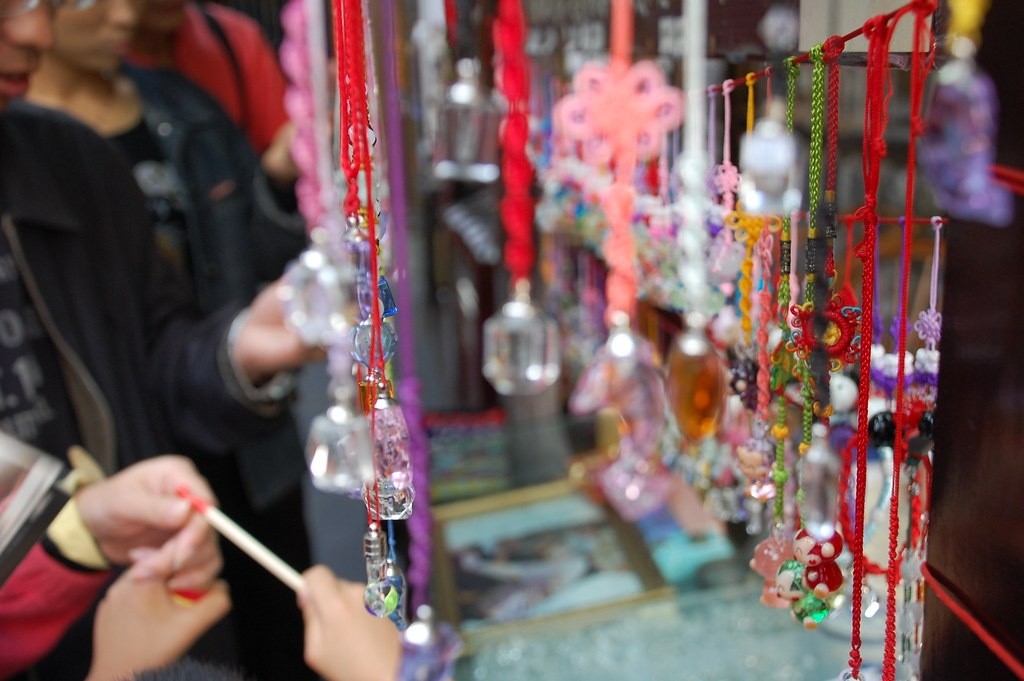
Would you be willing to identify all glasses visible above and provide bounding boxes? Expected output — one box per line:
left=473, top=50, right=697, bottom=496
left=0, top=0, right=96, bottom=18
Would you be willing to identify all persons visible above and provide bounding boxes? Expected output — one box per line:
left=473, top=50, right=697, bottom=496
left=29, top=1, right=309, bottom=559
left=0, top=426, right=227, bottom=680
left=0, top=2, right=362, bottom=676
left=292, top=564, right=406, bottom=680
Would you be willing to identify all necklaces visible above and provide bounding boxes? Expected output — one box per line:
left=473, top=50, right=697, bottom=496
left=472, top=5, right=946, bottom=676
left=279, top=0, right=436, bottom=650
